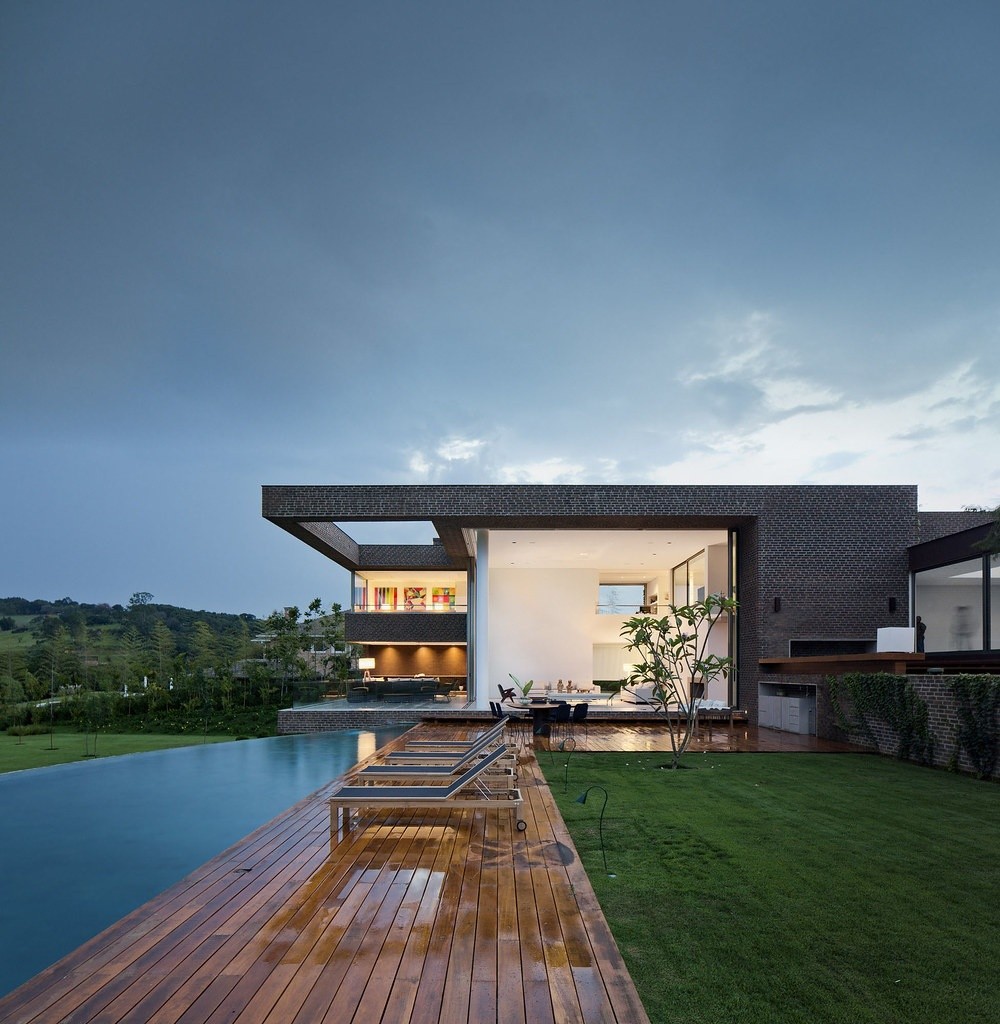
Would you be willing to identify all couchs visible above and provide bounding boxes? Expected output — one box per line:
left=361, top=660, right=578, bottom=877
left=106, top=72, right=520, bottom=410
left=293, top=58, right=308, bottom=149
left=345, top=678, right=440, bottom=701
left=621, top=681, right=663, bottom=705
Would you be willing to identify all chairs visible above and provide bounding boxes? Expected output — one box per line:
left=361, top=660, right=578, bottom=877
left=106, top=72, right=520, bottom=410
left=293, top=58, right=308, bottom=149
left=432, top=680, right=459, bottom=702
left=497, top=684, right=517, bottom=703
left=319, top=699, right=589, bottom=833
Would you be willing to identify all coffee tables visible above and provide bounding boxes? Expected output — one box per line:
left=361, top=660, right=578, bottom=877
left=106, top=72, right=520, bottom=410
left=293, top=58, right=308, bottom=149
left=383, top=693, right=434, bottom=702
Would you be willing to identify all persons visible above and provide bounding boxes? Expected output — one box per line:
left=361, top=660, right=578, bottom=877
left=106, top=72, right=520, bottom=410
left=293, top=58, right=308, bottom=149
left=916, top=616, right=926, bottom=653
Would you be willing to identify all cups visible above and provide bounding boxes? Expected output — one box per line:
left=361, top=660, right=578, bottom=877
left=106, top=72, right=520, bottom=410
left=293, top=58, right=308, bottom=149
left=459, top=685, right=464, bottom=691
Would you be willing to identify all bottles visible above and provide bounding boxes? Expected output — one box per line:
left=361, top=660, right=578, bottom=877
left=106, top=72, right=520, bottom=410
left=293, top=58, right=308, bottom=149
left=557, top=678, right=564, bottom=693
left=565, top=680, right=573, bottom=693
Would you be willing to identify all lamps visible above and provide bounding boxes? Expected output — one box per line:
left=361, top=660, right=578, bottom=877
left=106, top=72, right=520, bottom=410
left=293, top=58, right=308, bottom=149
left=537, top=723, right=555, bottom=766
left=359, top=658, right=376, bottom=684
left=558, top=737, right=576, bottom=790
left=576, top=785, right=608, bottom=872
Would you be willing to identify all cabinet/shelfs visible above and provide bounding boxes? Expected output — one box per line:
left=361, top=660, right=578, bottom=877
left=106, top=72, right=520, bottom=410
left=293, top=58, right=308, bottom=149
left=646, top=577, right=669, bottom=614
left=760, top=695, right=816, bottom=735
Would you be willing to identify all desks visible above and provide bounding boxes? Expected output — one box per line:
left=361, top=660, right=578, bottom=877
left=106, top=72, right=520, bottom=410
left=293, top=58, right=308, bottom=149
left=508, top=702, right=576, bottom=737
left=548, top=693, right=615, bottom=706
left=676, top=707, right=735, bottom=738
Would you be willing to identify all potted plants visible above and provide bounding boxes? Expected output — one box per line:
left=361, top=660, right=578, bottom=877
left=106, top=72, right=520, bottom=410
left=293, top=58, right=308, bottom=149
left=508, top=673, right=534, bottom=705
